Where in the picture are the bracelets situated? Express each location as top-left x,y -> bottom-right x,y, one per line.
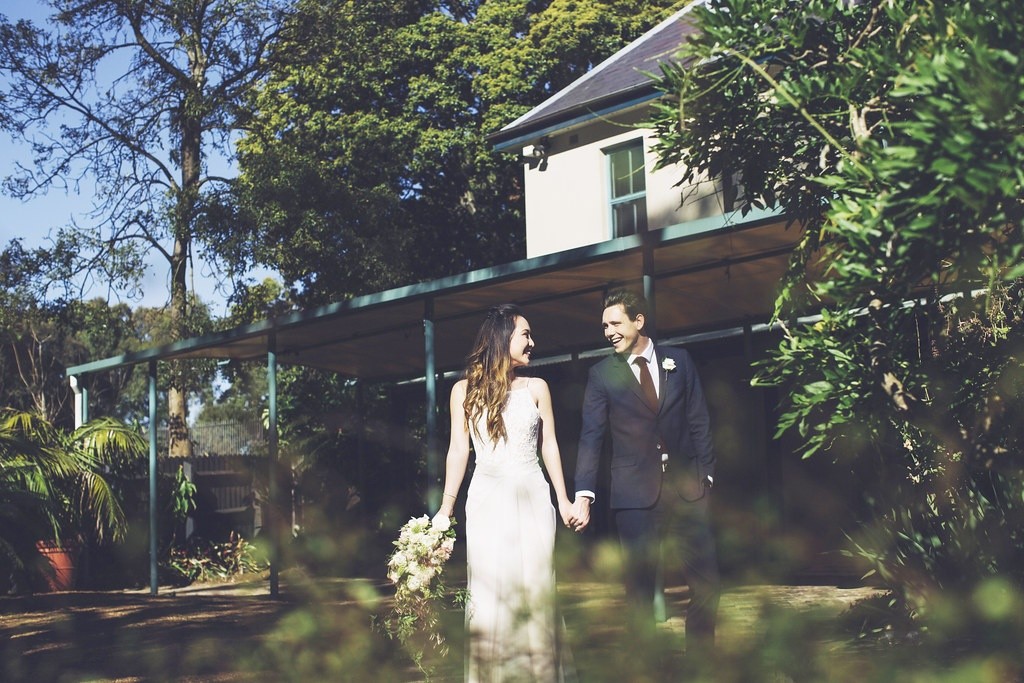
442,492 -> 457,500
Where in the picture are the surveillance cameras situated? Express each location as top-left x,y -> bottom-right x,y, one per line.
522,144 -> 545,158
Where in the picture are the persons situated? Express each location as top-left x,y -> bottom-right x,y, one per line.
432,305 -> 576,682
567,290 -> 722,660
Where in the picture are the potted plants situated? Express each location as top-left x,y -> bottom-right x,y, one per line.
5,317 -> 84,593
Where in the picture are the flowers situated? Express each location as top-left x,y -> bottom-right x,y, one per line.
662,357 -> 676,383
369,513 -> 469,683
